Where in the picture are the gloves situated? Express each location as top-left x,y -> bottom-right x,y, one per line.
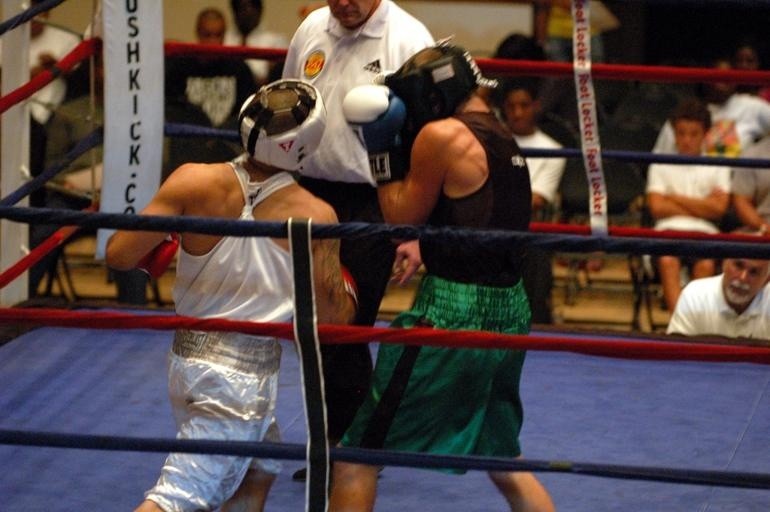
339,262 -> 361,308
343,85 -> 408,187
136,233 -> 180,279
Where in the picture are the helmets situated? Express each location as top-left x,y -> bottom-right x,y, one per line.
239,76 -> 327,174
384,40 -> 503,132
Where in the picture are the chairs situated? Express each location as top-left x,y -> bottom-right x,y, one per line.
538,127 -> 650,332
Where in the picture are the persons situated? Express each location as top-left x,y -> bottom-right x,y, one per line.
322,43 -> 556,511
644,52 -> 769,344
505,2 -> 618,317
28,0 -> 84,208
278,0 -> 439,481
103,78 -> 361,511
164,0 -> 288,169
